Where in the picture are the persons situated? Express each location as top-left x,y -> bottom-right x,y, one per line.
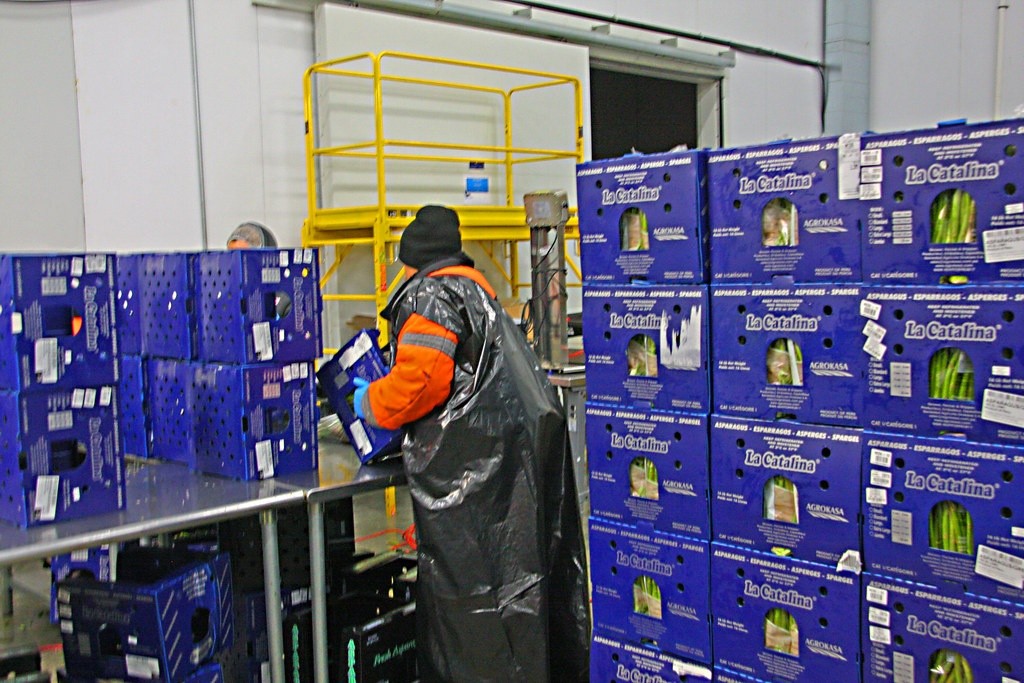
353,206 -> 592,683
227,218 -> 278,253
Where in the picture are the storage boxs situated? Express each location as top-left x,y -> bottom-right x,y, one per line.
0,248 -> 422,683
576,119 -> 1024,683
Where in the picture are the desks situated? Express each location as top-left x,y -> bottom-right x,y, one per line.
0,438 -> 411,683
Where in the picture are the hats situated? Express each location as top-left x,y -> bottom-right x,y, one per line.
398,206 -> 462,270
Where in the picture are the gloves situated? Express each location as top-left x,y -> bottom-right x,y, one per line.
352,378 -> 370,419
